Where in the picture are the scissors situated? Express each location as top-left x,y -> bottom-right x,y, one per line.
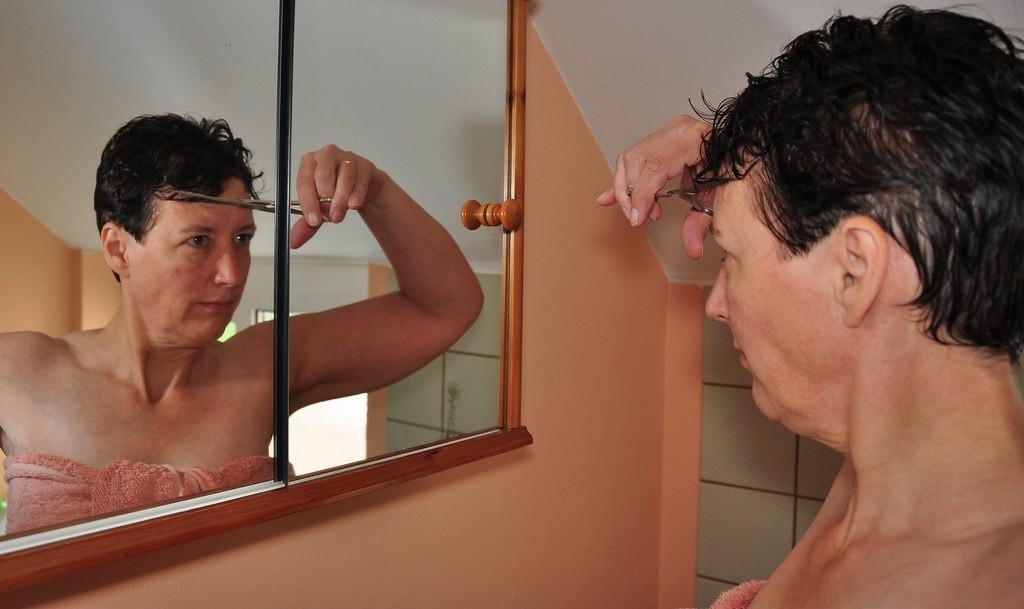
622,163 -> 733,218
166,187 -> 333,221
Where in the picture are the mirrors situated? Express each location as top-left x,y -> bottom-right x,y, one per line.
0,0 -> 536,595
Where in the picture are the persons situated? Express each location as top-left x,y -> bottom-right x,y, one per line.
1,113 -> 484,539
596,4 -> 1024,609
